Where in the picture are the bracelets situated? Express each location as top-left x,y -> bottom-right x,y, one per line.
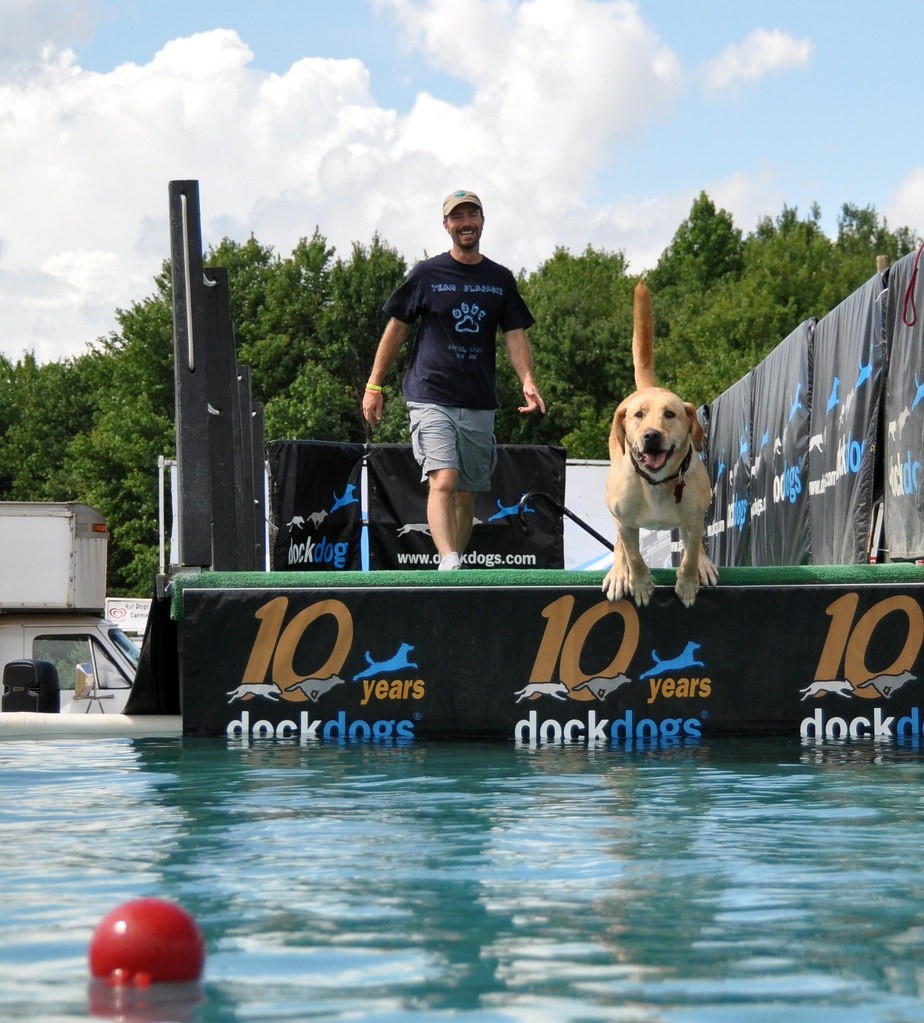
365,389 -> 381,395
367,384 -> 382,390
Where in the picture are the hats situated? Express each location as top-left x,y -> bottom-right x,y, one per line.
443,190 -> 483,218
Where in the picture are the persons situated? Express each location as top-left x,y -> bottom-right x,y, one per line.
362,190 -> 545,571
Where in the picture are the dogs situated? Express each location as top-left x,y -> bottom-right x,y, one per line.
601,277 -> 720,610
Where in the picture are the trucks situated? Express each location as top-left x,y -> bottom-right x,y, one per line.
1,500 -> 143,713
106,597 -> 152,650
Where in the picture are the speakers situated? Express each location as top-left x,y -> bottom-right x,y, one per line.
1,659 -> 60,714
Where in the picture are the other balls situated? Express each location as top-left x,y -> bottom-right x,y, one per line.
88,897 -> 203,989
